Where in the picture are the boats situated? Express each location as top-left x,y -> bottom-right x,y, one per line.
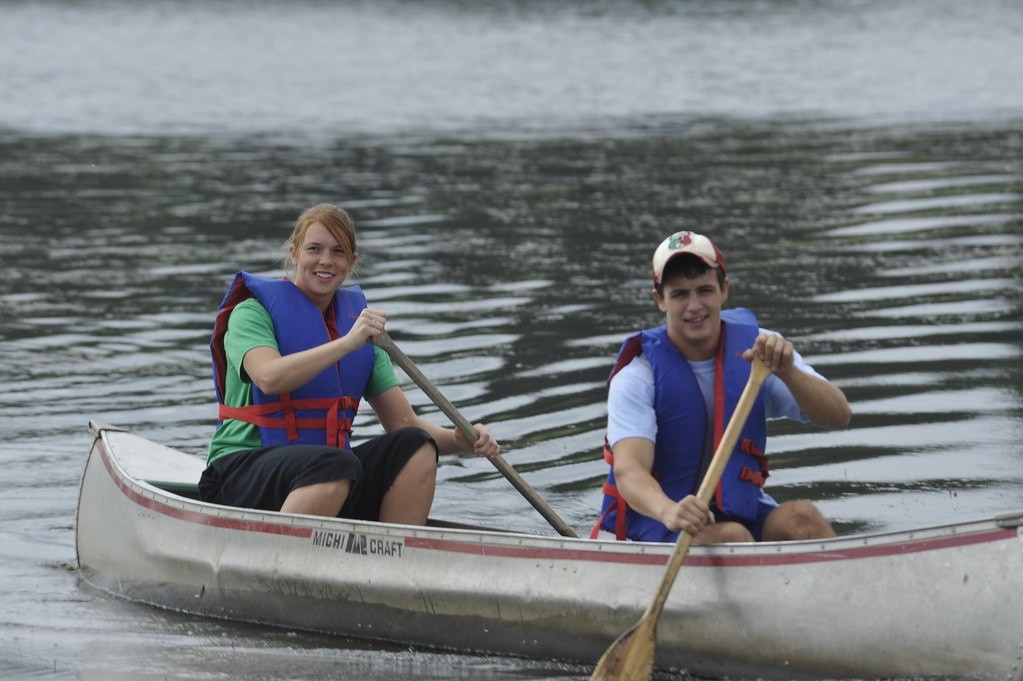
74,420 -> 1022,681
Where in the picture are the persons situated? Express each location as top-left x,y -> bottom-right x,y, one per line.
197,203 -> 501,525
596,231 -> 852,546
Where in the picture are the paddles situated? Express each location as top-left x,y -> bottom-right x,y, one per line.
587,345 -> 776,680
367,324 -> 581,540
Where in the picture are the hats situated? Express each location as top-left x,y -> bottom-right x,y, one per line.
651,231 -> 726,288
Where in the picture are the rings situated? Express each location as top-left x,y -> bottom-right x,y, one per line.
372,320 -> 376,326
